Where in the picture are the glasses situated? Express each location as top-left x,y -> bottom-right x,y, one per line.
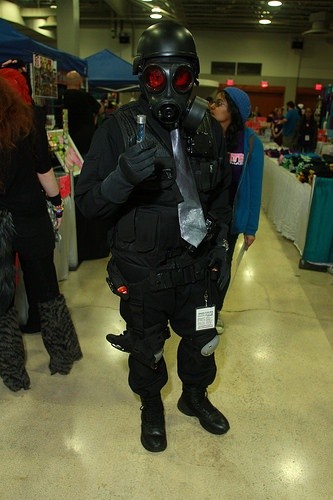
208,99 -> 229,110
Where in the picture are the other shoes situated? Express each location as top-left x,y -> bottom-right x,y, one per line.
19,321 -> 43,334
176,383 -> 230,434
141,391 -> 167,452
214,310 -> 224,334
107,332 -> 130,352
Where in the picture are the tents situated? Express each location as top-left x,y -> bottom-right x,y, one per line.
79,47 -> 146,105
0,18 -> 90,95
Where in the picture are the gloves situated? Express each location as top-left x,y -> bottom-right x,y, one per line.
208,222 -> 230,289
102,135 -> 156,203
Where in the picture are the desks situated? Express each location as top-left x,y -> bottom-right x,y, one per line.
261,141 -> 333,274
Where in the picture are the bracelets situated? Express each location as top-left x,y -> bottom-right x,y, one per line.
46,192 -> 65,213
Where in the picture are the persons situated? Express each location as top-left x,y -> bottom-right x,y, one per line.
1,56 -> 122,135
201,80 -> 333,159
0,67 -> 48,335
0,79 -> 83,394
54,70 -> 106,156
73,20 -> 234,452
207,85 -> 264,336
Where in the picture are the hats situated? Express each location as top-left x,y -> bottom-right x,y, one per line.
223,86 -> 251,122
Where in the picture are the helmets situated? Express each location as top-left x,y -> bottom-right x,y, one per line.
132,21 -> 200,74
